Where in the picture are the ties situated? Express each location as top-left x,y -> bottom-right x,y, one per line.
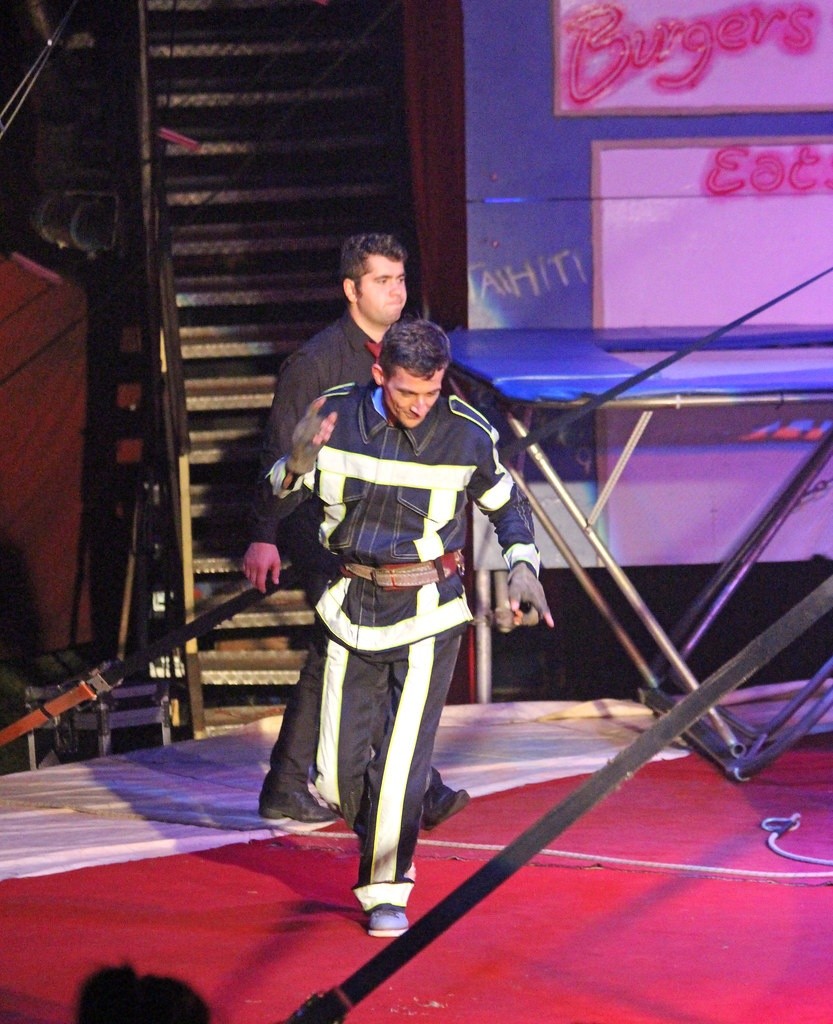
367,341 -> 380,364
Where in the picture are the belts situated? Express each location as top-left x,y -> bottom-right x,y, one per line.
341,547 -> 465,589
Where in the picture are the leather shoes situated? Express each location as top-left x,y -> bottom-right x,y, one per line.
422,785 -> 471,832
257,788 -> 336,822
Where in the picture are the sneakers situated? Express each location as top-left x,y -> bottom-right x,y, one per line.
368,903 -> 408,937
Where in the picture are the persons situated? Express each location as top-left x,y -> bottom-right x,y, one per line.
240,231 -> 471,831
247,318 -> 555,938
